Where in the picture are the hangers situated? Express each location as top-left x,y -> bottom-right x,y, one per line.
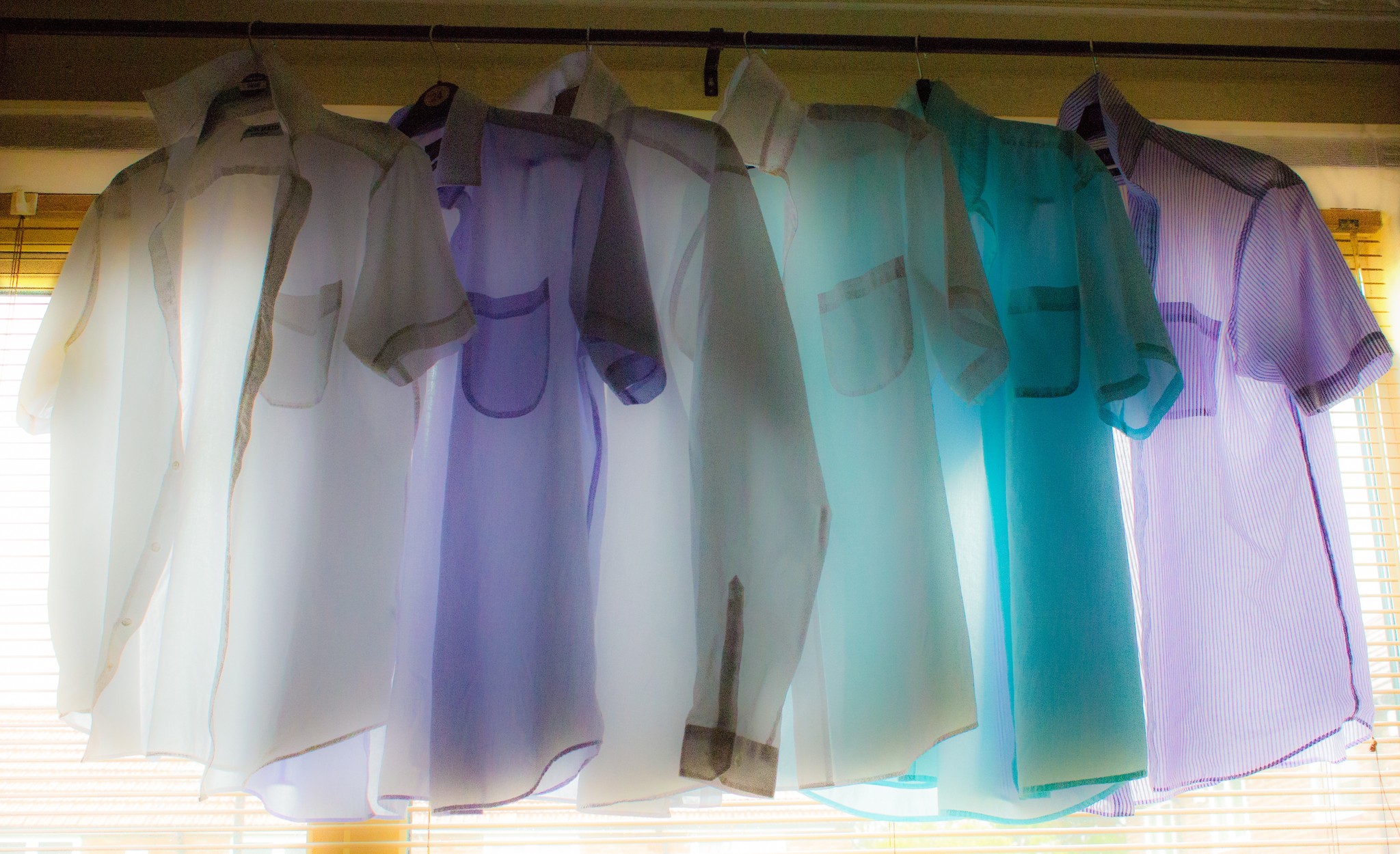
393,22 -> 615,153
112,19 -> 275,183
